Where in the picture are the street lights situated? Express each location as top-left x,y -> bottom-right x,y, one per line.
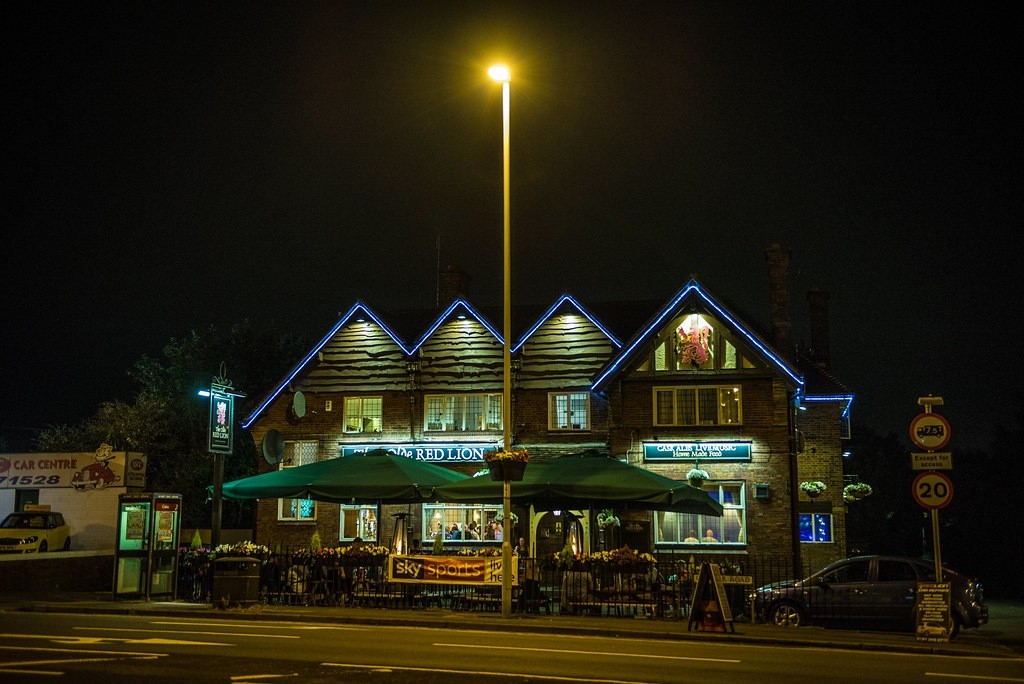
197,362 -> 247,602
487,65 -> 512,620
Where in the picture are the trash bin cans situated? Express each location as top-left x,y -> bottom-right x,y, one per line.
211,557 -> 262,610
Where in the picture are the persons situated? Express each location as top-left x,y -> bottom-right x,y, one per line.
437,520 -> 503,540
684,530 -> 699,542
702,529 -> 719,542
411,539 -> 419,550
352,537 -> 363,546
514,538 -> 530,557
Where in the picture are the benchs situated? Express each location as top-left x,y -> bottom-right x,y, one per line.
570,602 -> 658,619
352,592 -> 552,615
264,590 -> 346,605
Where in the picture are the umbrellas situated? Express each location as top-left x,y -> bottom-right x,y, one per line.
206,448 -> 472,545
435,451 -> 724,557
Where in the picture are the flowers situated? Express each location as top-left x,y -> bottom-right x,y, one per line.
459,547 -> 519,556
553,545 -> 654,562
494,510 -> 519,523
686,469 -> 709,480
842,484 -> 872,500
598,508 -> 620,528
293,543 -> 389,555
801,481 -> 827,490
180,540 -> 269,554
484,447 -> 531,462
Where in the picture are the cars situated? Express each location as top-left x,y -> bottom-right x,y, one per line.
743,555 -> 989,640
0,505 -> 71,554
917,622 -> 947,637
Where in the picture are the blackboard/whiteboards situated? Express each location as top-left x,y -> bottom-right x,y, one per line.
688,563 -> 733,622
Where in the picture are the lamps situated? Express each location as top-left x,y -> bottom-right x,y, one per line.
458,308 -> 466,320
842,449 -> 851,457
356,312 -> 365,323
552,509 -> 561,516
798,404 -> 807,411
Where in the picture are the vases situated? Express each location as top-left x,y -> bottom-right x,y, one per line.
487,460 -> 527,481
604,525 -> 614,531
691,477 -> 703,487
804,489 -> 821,498
544,561 -> 650,573
849,490 -> 868,497
179,551 -> 268,560
501,523 -> 516,529
292,556 -> 386,566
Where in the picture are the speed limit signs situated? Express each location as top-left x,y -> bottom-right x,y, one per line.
912,471 -> 953,509
